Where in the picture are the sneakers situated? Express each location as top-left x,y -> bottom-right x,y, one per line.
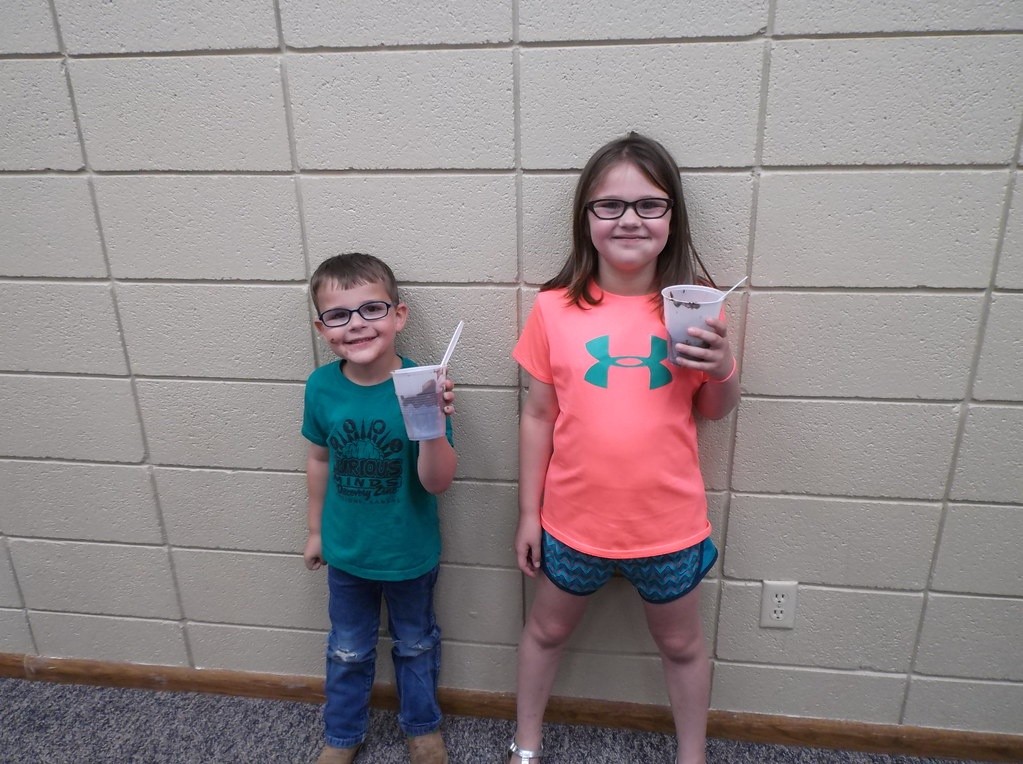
316,745 -> 357,764
405,730 -> 447,764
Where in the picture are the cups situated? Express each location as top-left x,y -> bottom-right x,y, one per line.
391,364 -> 448,441
661,285 -> 726,366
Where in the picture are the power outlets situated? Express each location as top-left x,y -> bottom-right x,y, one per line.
759,579 -> 798,629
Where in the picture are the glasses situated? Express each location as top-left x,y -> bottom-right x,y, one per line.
584,197 -> 673,220
319,301 -> 399,327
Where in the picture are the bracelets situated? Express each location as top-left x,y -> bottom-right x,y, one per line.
707,355 -> 736,385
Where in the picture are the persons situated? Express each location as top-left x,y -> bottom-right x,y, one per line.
302,252 -> 462,764
509,130 -> 740,764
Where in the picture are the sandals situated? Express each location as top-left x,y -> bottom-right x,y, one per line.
507,736 -> 543,764
